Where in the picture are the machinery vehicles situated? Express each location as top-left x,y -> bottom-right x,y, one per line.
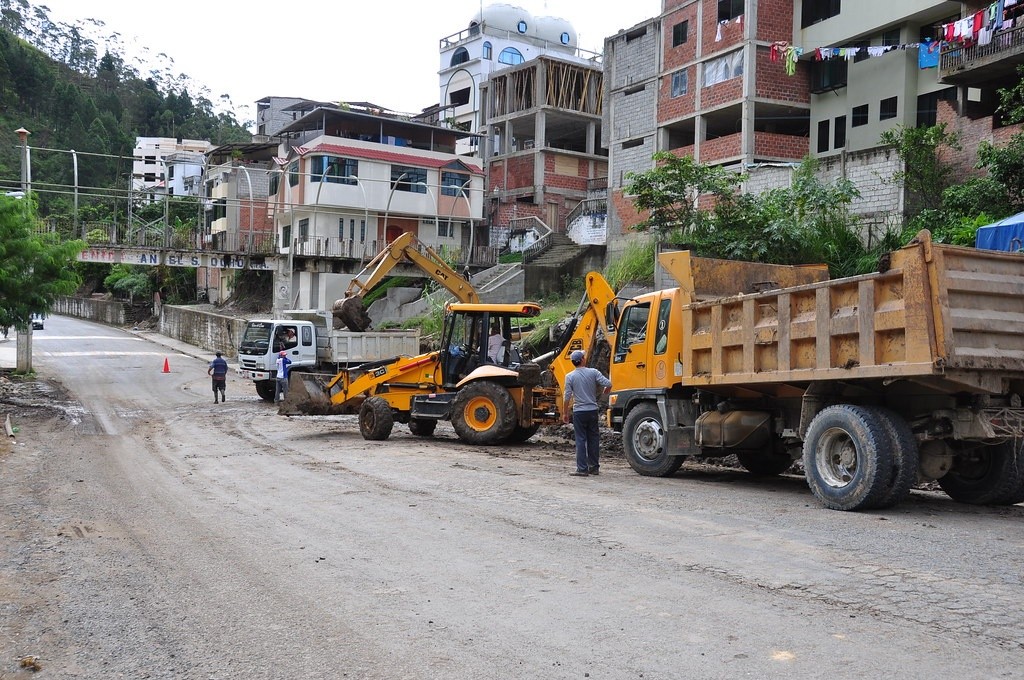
276,231 -> 620,446
237,319 -> 421,400
606,228 -> 1024,512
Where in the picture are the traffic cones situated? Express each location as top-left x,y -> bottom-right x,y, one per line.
160,358 -> 171,374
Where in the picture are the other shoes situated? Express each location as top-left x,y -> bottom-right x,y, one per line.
588,468 -> 599,475
569,470 -> 589,477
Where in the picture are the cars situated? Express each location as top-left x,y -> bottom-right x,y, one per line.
31,314 -> 44,330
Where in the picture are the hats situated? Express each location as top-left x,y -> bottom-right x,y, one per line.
571,350 -> 585,362
280,351 -> 287,355
216,350 -> 221,355
288,328 -> 296,334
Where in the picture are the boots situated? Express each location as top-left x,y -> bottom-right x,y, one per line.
221,389 -> 225,402
213,392 -> 218,404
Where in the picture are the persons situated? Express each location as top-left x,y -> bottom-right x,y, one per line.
563,350 -> 612,476
458,325 -> 507,380
208,350 -> 228,404
274,351 -> 291,403
286,328 -> 296,342
